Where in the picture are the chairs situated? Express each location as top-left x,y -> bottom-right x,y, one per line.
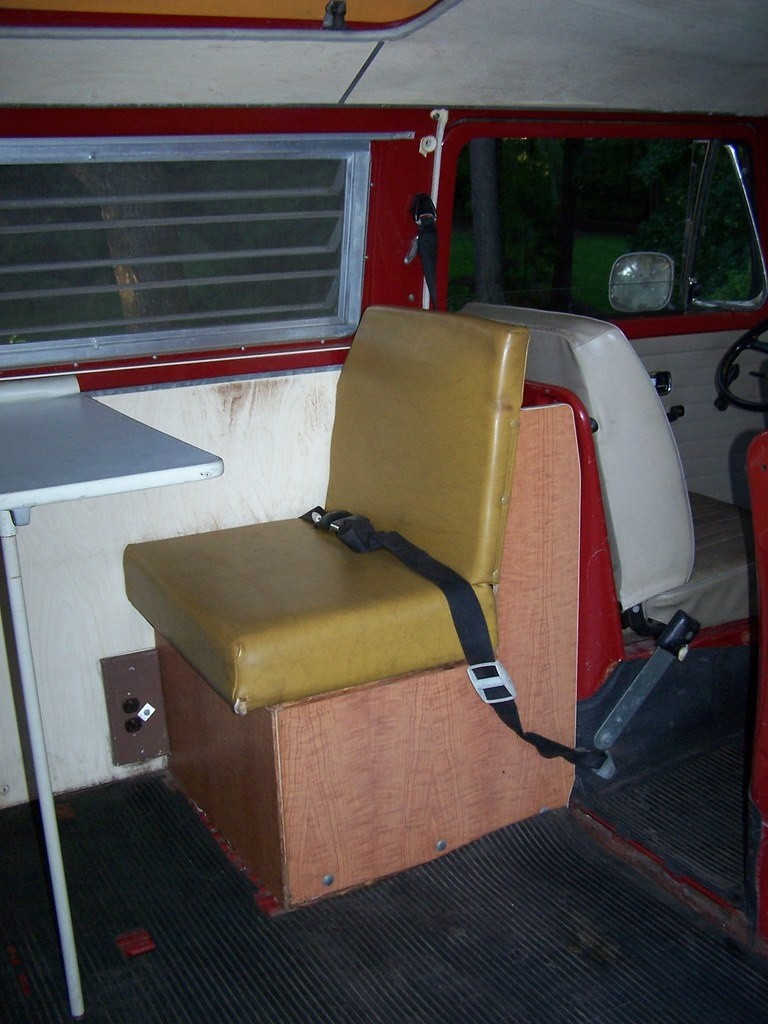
124,301 -> 767,911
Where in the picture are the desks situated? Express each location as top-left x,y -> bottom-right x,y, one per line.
0,393 -> 225,1023
